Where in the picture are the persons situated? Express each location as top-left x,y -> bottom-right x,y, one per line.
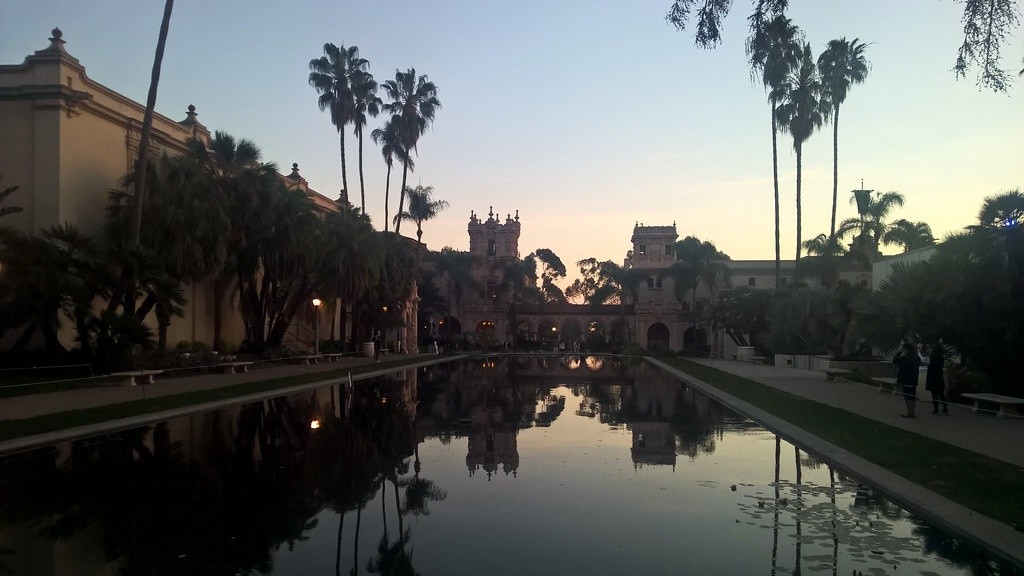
893,342 -> 920,418
540,336 -> 584,353
372,330 -> 383,363
504,338 -> 511,352
925,343 -> 948,415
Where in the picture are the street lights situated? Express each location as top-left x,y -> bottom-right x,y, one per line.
311,390 -> 320,429
552,327 -> 556,352
312,299 -> 321,354
430,317 -> 433,337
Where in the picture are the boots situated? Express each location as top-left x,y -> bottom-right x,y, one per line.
942,403 -> 948,412
931,404 -> 938,413
901,400 -> 916,418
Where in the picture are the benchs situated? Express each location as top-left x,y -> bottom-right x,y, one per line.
379,348 -> 390,354
298,355 -> 323,365
110,369 -> 164,386
218,361 -> 254,374
961,393 -> 1024,419
322,352 -> 345,362
820,368 -> 852,382
873,377 -> 899,394
751,356 -> 767,364
